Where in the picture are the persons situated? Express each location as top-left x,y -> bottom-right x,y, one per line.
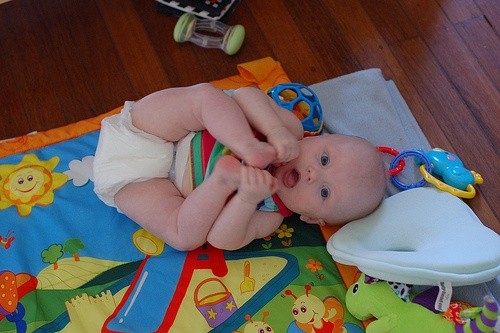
92,82 -> 387,251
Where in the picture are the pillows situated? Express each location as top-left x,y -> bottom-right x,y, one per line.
326,187 -> 500,287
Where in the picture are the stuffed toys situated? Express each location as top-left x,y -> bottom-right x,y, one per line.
345,272 -> 499,333
